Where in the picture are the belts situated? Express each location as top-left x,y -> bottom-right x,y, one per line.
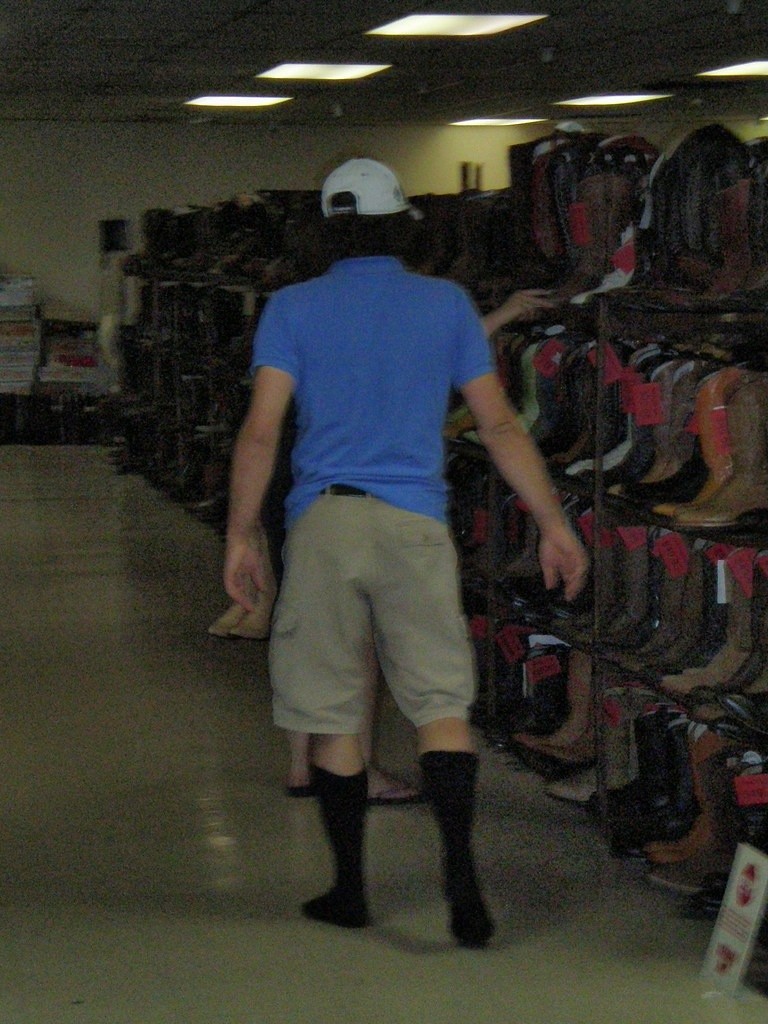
322,483 -> 368,497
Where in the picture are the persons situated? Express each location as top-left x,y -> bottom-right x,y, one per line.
280,288 -> 552,804
224,156 -> 589,952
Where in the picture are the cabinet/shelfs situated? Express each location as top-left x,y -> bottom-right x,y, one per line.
120,265 -> 768,998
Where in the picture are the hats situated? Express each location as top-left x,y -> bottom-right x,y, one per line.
319,154 -> 412,216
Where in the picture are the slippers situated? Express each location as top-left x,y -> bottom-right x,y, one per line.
367,768 -> 426,804
286,777 -> 318,797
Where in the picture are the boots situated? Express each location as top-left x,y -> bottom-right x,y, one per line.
302,762 -> 372,928
106,190 -> 327,641
420,750 -> 495,950
410,132 -> 768,989
